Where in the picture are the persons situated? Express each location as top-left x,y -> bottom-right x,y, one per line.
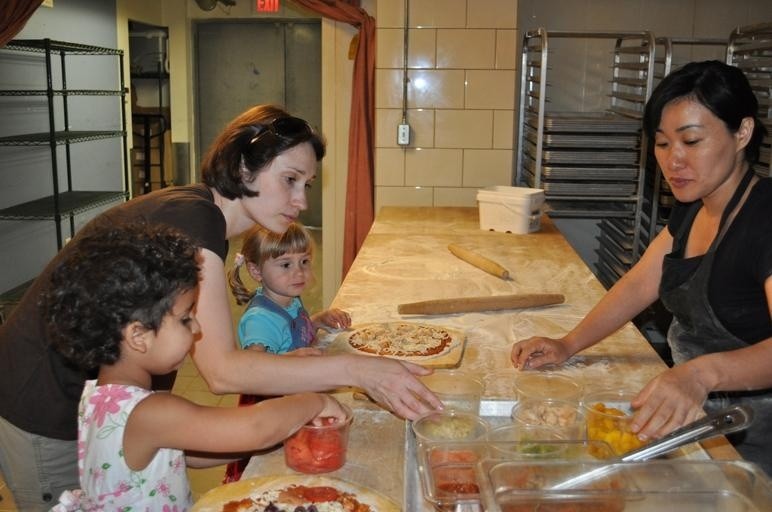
510,58 -> 772,485
226,218 -> 355,482
0,100 -> 448,512
42,213 -> 349,512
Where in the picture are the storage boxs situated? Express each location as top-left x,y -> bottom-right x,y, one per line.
477,183 -> 546,236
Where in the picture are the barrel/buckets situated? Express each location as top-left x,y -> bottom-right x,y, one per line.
474,183 -> 544,235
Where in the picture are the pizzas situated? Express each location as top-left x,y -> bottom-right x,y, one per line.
198,475 -> 380,512
347,321 -> 461,360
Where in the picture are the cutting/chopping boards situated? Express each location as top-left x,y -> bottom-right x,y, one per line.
331,319 -> 469,369
189,473 -> 404,511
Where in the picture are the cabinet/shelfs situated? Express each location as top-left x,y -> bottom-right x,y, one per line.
641,23 -> 772,261
2,35 -> 134,309
519,28 -> 655,294
